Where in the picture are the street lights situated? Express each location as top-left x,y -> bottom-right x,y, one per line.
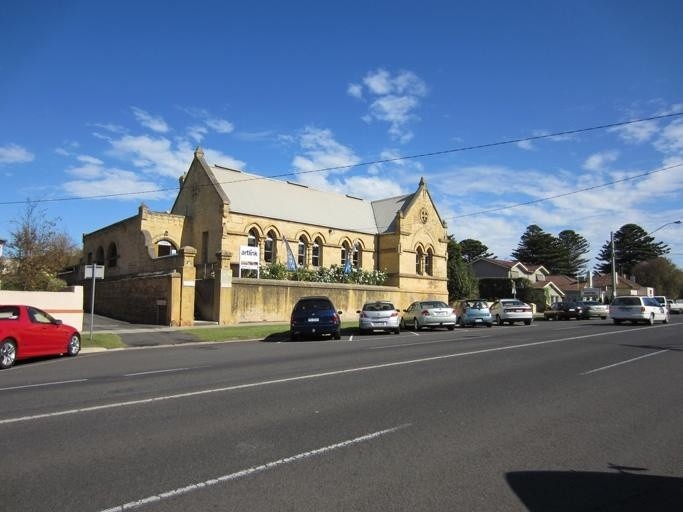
609,220 -> 682,300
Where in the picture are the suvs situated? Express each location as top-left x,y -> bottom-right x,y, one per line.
609,295 -> 682,325
544,301 -> 588,321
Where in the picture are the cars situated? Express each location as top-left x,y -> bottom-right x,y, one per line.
356,301 -> 401,334
401,301 -> 457,331
0,304 -> 82,368
454,299 -> 492,328
489,298 -> 533,325
584,302 -> 609,320
290,296 -> 342,341
